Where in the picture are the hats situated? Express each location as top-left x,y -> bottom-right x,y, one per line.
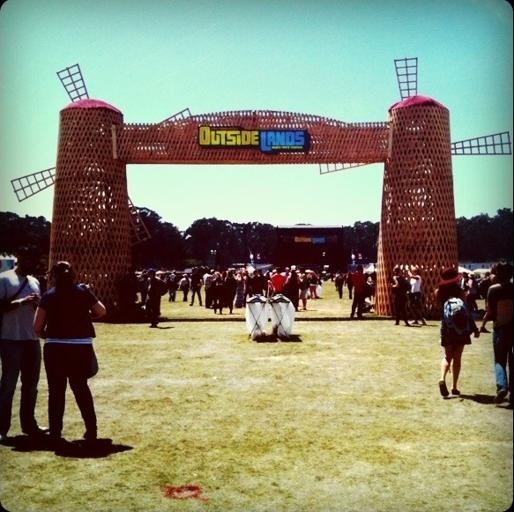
439,267 -> 464,286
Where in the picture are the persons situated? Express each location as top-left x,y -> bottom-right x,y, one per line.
32,260 -> 106,443
434,258 -> 513,406
121,262 -> 428,327
0,249 -> 48,441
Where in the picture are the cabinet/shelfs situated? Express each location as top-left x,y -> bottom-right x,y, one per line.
246,294 -> 291,339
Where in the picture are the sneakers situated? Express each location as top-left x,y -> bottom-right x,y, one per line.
437,380 -> 514,405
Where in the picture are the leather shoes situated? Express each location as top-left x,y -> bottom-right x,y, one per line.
0,431 -> 8,443
23,426 -> 50,434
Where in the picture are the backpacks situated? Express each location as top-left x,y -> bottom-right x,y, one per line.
444,297 -> 472,339
493,298 -> 514,329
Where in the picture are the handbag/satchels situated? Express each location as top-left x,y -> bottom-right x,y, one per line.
88,345 -> 99,378
